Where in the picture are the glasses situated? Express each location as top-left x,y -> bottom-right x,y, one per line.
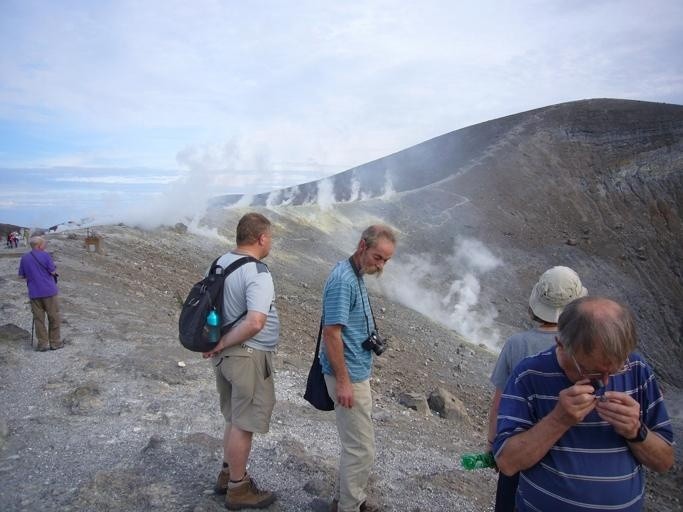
566,346 -> 632,380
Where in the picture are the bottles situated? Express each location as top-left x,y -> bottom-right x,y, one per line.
207,311 -> 220,343
462,453 -> 498,471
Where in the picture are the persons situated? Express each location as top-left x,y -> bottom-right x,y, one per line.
204,211 -> 280,512
18,236 -> 67,352
489,296 -> 676,511
6,232 -> 19,249
317,224 -> 398,511
487,265 -> 591,512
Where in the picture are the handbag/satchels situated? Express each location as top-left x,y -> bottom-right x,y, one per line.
303,309 -> 338,413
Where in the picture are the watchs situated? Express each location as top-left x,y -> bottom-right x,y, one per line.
627,421 -> 647,444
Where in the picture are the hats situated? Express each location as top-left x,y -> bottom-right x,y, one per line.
529,266 -> 588,323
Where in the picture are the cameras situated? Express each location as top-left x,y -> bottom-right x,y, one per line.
362,332 -> 386,356
588,379 -> 605,395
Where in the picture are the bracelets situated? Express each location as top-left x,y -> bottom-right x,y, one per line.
488,439 -> 493,445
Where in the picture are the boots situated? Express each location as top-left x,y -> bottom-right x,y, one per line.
225,478 -> 276,510
214,471 -> 229,493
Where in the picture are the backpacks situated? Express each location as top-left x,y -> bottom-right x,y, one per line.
177,254 -> 273,354
